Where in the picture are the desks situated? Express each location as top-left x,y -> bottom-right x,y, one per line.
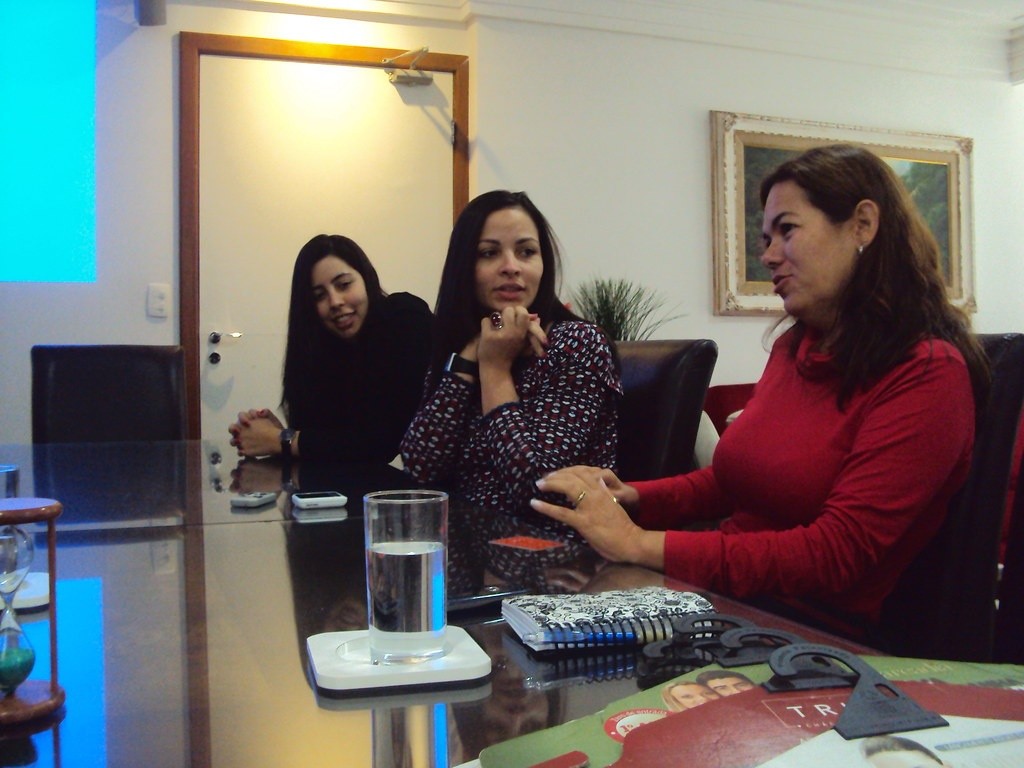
0,444 -> 895,767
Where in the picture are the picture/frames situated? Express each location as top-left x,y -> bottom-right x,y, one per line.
710,110 -> 977,320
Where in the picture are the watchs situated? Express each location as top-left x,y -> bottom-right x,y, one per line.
280,428 -> 296,453
443,353 -> 479,377
280,463 -> 299,493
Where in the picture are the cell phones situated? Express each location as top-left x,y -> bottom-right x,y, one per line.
292,490 -> 347,509
447,582 -> 527,611
291,508 -> 349,523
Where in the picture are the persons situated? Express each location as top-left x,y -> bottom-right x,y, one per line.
228,235 -> 434,490
532,143 -> 992,643
859,736 -> 952,768
696,671 -> 755,698
230,457 -> 394,684
661,681 -> 723,713
399,191 -> 623,548
375,489 -> 612,758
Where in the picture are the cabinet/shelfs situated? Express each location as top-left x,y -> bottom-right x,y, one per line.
0,496 -> 68,723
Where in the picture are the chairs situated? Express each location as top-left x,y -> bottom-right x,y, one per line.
606,340 -> 717,488
875,331 -> 1024,665
31,343 -> 189,444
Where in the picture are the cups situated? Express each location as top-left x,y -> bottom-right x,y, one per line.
365,490 -> 450,665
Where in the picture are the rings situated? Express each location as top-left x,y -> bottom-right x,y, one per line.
573,492 -> 585,506
490,311 -> 503,330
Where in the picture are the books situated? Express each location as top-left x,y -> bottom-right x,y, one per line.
500,585 -> 719,651
